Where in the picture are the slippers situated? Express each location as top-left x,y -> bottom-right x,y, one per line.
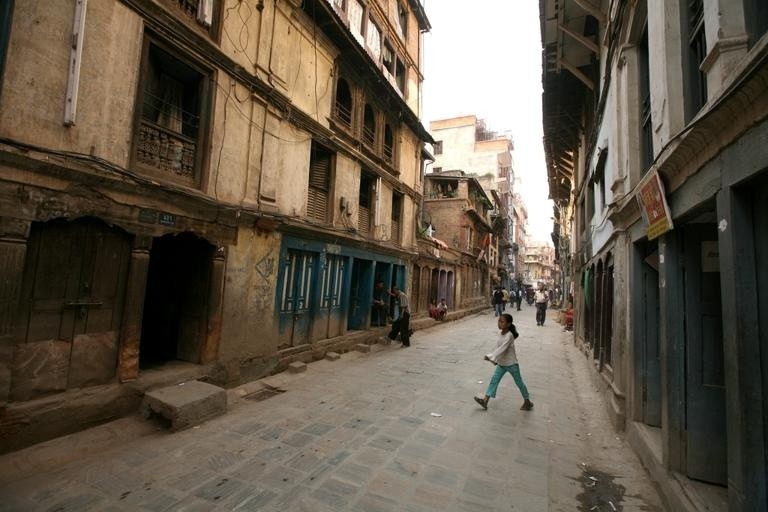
520,403 -> 533,410
474,397 -> 487,408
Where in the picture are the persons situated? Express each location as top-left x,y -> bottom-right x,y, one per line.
429,295 -> 443,320
490,281 -> 574,331
386,285 -> 412,348
437,297 -> 448,317
473,313 -> 534,411
371,279 -> 388,323
